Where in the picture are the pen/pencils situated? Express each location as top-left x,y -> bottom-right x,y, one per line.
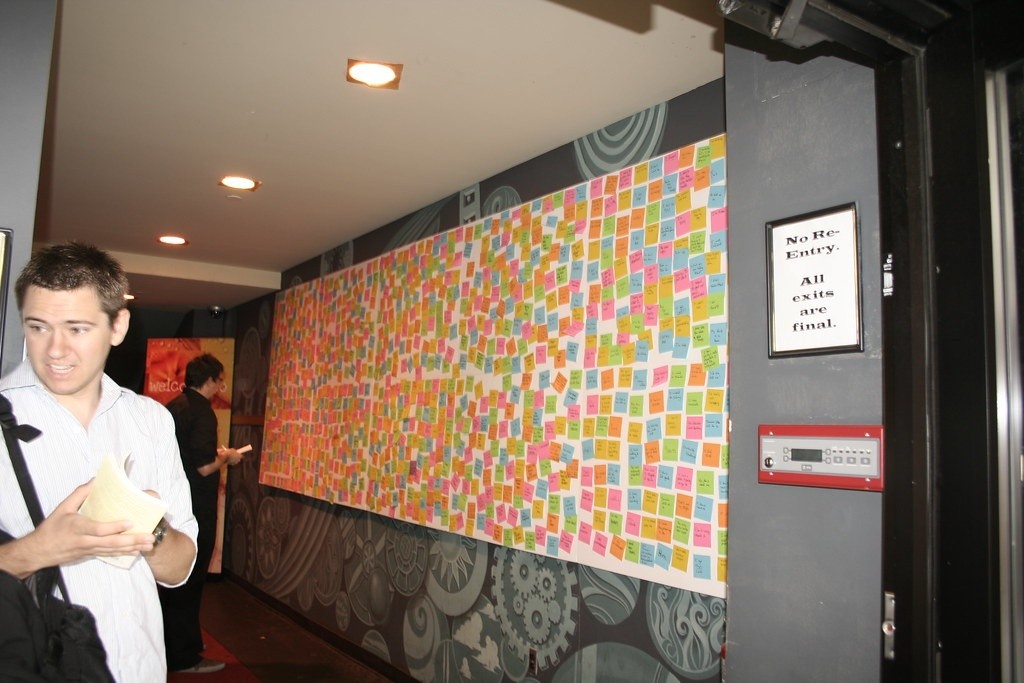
221,444 -> 227,451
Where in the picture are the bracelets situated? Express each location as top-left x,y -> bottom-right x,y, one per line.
155,519 -> 165,544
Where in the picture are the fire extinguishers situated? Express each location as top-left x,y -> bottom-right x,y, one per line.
721,618 -> 726,683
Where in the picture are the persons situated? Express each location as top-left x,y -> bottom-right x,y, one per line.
160,353 -> 246,673
0,241 -> 199,683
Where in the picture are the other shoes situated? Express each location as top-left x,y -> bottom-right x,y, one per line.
176,658 -> 225,673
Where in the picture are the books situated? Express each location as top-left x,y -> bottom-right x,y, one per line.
236,444 -> 252,454
78,453 -> 166,569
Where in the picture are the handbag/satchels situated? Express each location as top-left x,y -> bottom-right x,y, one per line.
0,568 -> 116,683
